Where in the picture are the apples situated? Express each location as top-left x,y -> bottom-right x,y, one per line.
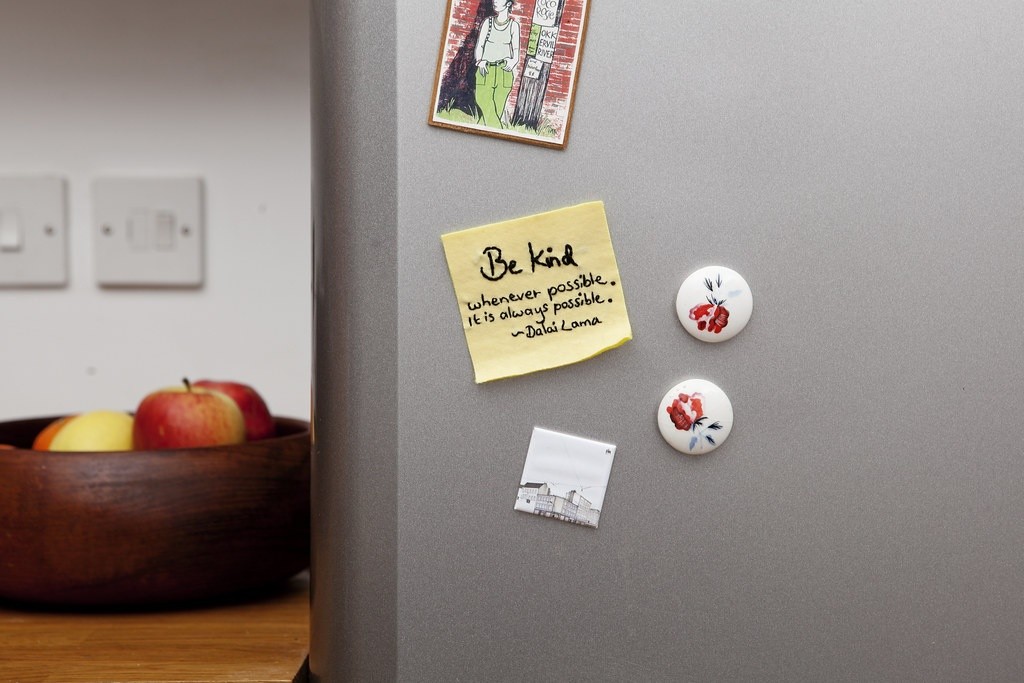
131,378 -> 271,450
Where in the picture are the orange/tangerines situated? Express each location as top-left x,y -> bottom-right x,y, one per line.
31,416 -> 75,453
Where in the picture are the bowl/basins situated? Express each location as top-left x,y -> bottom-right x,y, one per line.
0,415 -> 313,609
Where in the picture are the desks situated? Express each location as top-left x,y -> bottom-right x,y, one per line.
0,572 -> 311,682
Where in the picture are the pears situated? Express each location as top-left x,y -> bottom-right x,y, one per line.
48,410 -> 133,452
